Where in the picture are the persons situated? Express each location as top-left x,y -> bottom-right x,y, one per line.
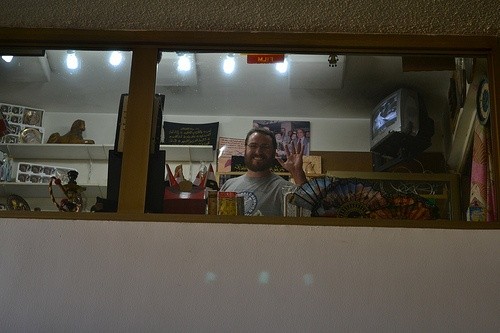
219,127 -> 308,217
254,122 -> 310,155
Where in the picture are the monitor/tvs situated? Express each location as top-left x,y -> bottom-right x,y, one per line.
369,88 -> 419,147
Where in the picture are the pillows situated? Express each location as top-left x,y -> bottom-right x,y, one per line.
163,122 -> 219,150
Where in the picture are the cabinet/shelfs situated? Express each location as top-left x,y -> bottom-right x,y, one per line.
1,141 -> 215,214
220,169 -> 461,224
